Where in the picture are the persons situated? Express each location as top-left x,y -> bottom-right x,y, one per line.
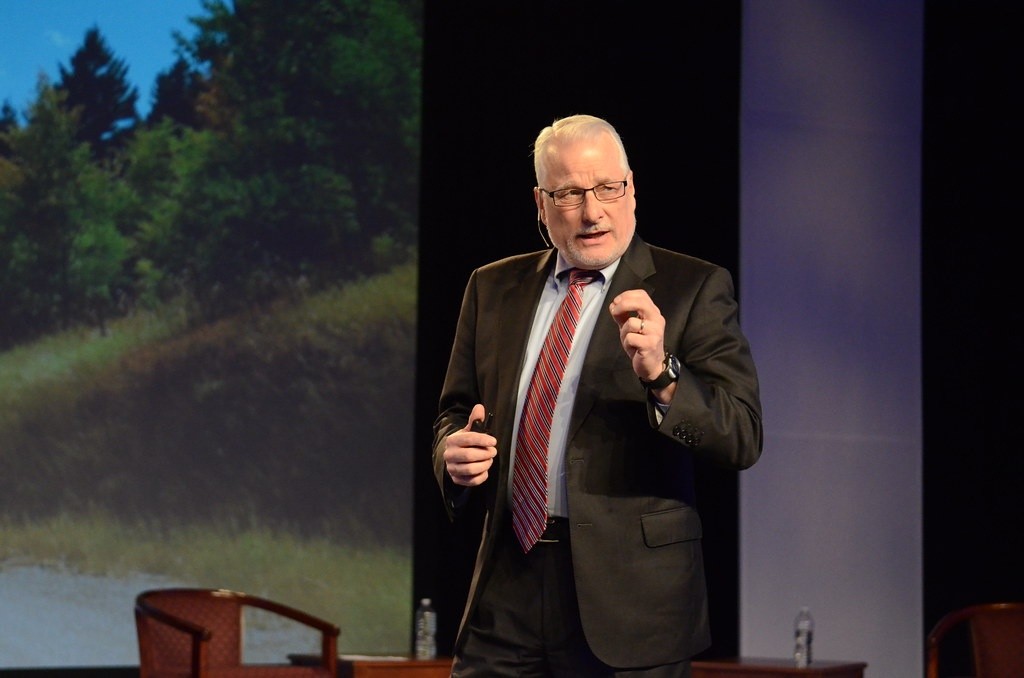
426,115 -> 763,678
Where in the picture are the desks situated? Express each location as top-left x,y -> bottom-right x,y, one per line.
690,656 -> 867,678
289,652 -> 454,678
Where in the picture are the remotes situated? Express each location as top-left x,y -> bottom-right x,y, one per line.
469,412 -> 495,449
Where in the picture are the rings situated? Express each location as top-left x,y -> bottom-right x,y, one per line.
639,318 -> 645,333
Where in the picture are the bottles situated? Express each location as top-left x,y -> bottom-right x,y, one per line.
414,598 -> 438,662
792,604 -> 815,668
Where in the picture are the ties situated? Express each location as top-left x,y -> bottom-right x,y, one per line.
510,270 -> 596,555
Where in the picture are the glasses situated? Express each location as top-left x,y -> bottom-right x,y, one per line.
538,173 -> 629,206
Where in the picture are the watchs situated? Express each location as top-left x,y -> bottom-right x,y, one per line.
638,352 -> 681,391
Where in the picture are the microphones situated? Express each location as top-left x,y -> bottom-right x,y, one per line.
537,207 -> 550,247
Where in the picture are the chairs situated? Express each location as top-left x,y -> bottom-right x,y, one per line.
134,586 -> 341,678
928,602 -> 1024,678
